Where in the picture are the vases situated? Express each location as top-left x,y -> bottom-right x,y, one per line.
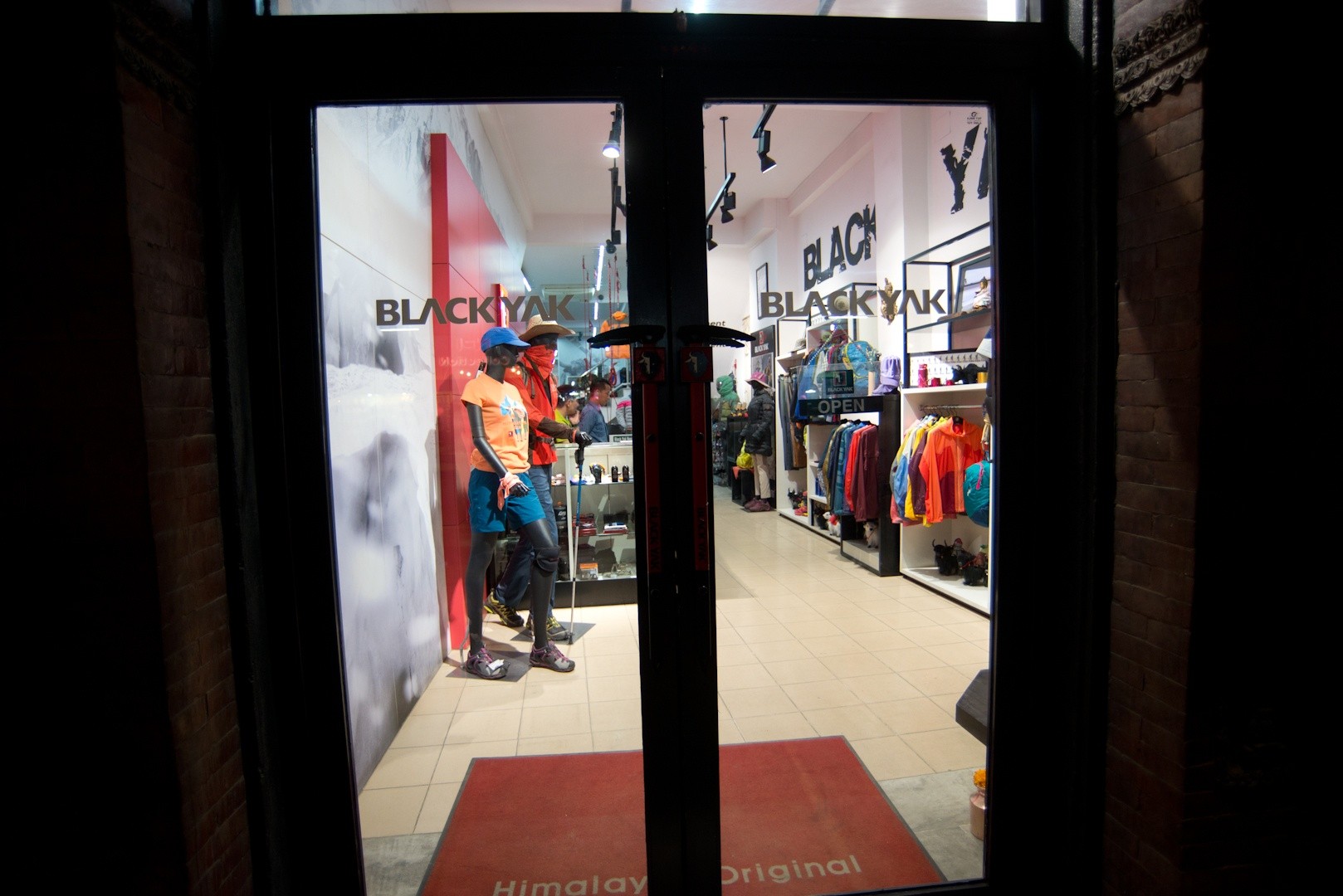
969,791 -> 985,839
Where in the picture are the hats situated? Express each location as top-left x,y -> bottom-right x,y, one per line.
518,314 -> 575,342
480,327 -> 531,352
746,371 -> 769,389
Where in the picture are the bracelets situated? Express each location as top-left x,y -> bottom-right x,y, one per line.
573,429 -> 577,443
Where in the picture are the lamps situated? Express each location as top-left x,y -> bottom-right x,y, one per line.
602,103 -> 777,255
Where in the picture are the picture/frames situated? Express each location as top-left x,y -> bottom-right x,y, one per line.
756,262 -> 769,319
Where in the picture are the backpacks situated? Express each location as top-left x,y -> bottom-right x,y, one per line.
962,450 -> 990,528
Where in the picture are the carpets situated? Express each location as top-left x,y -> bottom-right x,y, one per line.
416,736 -> 949,896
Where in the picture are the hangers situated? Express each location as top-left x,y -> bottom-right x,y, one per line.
922,405 -> 964,425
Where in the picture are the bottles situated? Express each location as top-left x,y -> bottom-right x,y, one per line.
918,364 -> 928,388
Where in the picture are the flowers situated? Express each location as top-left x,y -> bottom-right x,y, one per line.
973,769 -> 986,788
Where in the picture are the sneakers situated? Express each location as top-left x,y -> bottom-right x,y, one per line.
466,645 -> 506,679
795,504 -> 808,516
749,500 -> 770,511
483,588 -> 525,627
528,640 -> 575,672
526,613 -> 568,640
745,497 -> 759,509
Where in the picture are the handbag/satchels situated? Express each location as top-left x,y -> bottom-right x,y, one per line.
736,440 -> 753,469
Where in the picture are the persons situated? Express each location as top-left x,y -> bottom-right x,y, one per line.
555,374 -> 632,443
714,371 -> 776,511
484,313 -> 594,641
460,327 -> 575,679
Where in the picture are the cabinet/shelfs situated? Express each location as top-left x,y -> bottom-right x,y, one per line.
775,282 -> 899,577
494,443 -> 638,584
900,222 -> 992,615
